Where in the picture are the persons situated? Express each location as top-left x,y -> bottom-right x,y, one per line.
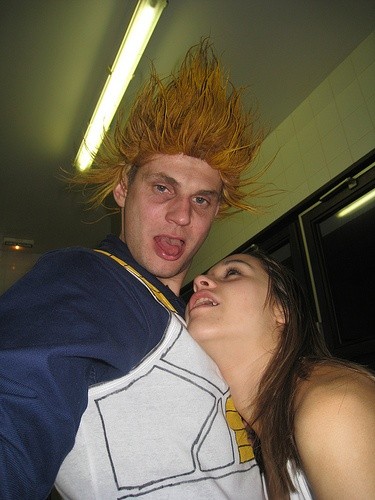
1,38 -> 317,500
187,243 -> 374,500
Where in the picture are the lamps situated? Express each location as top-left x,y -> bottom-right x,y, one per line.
73,0 -> 168,173
